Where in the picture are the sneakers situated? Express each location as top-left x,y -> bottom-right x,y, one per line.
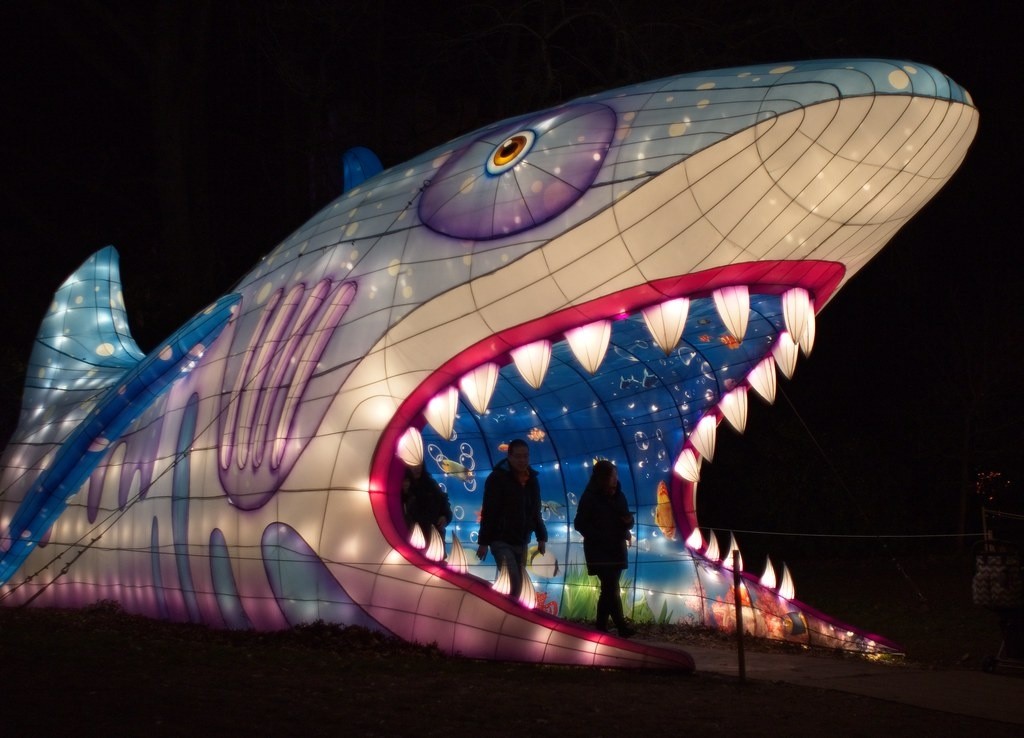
619,625 -> 635,637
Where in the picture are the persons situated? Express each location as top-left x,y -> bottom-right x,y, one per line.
475,440 -> 548,603
399,458 -> 452,561
574,460 -> 639,640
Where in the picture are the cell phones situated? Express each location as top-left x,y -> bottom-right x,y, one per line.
624,511 -> 636,518
